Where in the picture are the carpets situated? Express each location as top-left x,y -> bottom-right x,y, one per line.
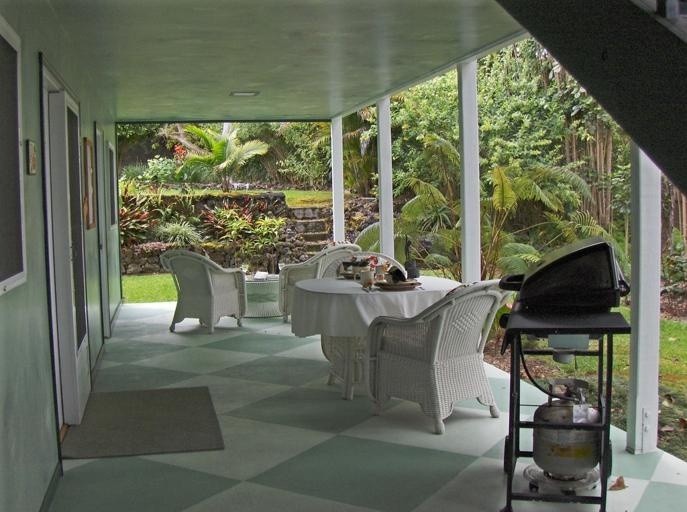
59,386 -> 225,460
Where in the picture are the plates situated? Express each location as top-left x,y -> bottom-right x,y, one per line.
340,271 -> 360,280
375,281 -> 421,290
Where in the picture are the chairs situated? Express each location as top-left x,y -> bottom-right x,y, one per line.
157,247 -> 247,335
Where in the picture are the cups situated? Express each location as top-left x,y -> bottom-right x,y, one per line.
354,270 -> 374,288
342,260 -> 370,274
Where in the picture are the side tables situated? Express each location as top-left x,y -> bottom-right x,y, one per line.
241,273 -> 285,317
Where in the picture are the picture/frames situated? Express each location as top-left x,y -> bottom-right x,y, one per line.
26,139 -> 37,175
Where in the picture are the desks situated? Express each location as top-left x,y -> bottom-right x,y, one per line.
495,310 -> 632,512
